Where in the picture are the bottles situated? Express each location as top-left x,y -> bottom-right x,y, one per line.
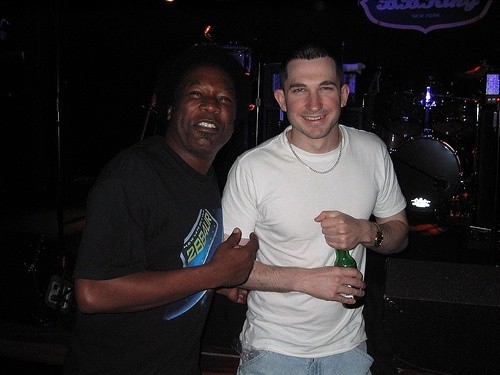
333,248 -> 368,309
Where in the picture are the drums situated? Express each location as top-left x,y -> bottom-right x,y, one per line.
389,131 -> 474,213
449,96 -> 483,124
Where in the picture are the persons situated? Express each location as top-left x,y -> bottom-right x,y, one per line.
58,55 -> 260,375
216,43 -> 410,375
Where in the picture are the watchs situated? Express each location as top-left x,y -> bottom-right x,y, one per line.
367,222 -> 384,249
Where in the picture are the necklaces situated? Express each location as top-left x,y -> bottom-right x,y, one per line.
286,128 -> 342,175
